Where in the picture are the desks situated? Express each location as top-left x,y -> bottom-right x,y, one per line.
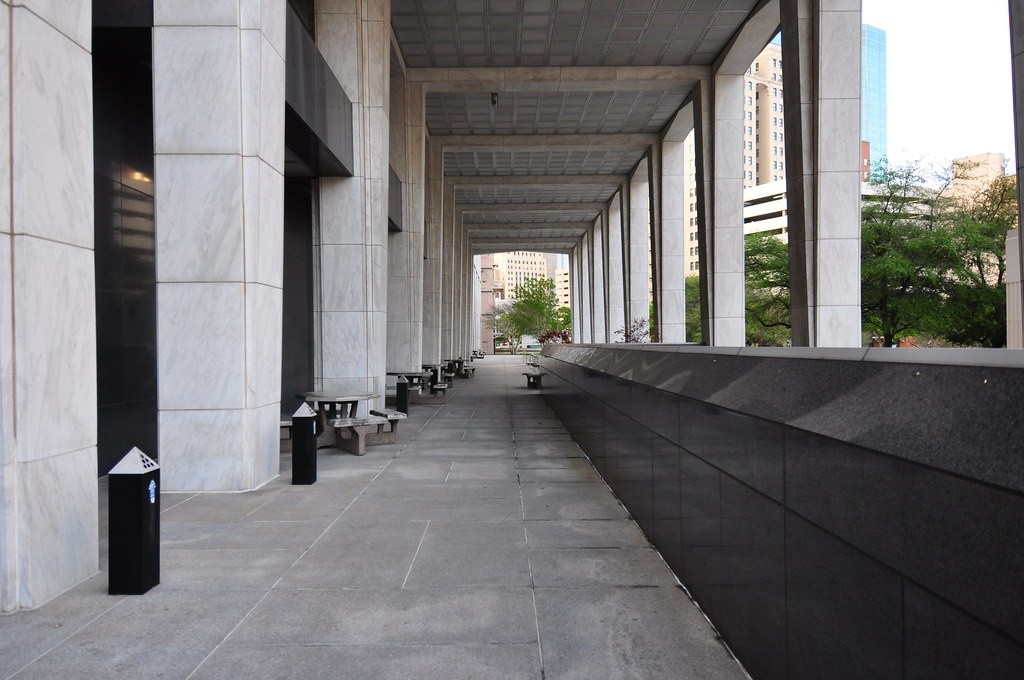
444,359 -> 469,376
295,392 -> 381,448
387,371 -> 433,405
423,364 -> 435,371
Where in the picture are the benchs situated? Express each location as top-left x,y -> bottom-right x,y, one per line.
522,372 -> 545,390
280,410 -> 409,456
385,350 -> 486,408
